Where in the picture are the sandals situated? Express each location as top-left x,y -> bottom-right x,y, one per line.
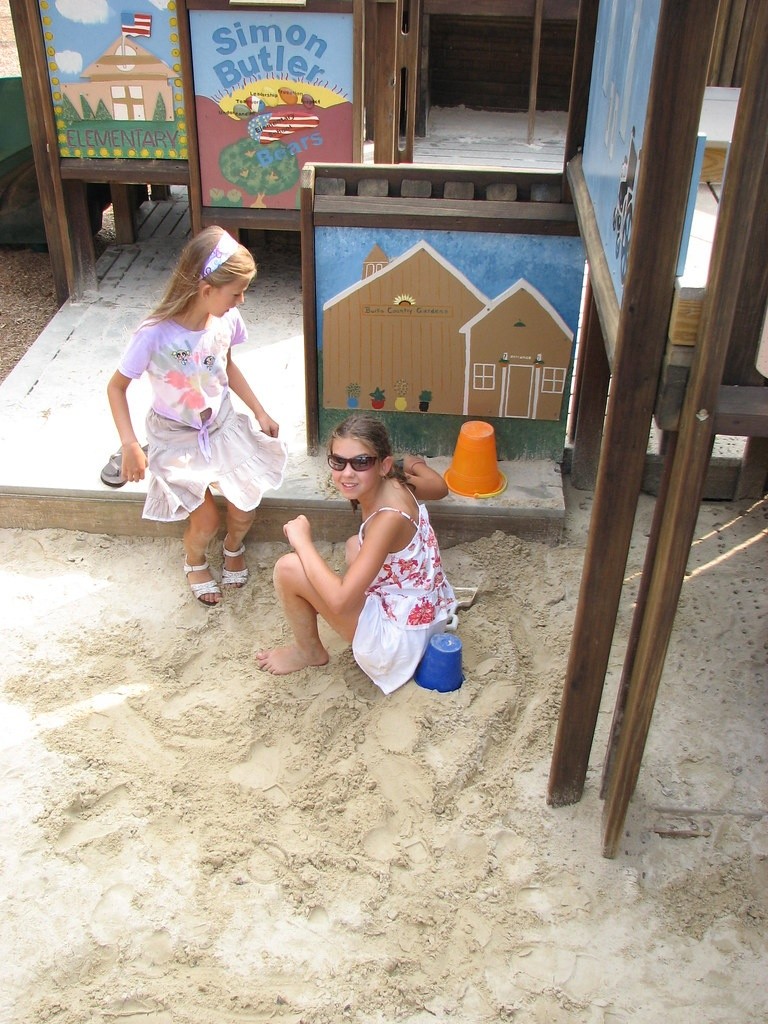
101,445 -> 149,487
222,533 -> 249,588
184,553 -> 222,606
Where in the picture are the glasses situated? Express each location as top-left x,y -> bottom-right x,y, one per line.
327,454 -> 378,472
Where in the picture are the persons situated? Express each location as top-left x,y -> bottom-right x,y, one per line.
106,226 -> 288,609
257,413 -> 458,696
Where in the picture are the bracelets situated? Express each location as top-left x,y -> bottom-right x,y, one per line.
409,460 -> 426,475
121,440 -> 138,448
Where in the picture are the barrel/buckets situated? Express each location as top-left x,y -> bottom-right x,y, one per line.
444,421 -> 508,498
414,633 -> 465,693
